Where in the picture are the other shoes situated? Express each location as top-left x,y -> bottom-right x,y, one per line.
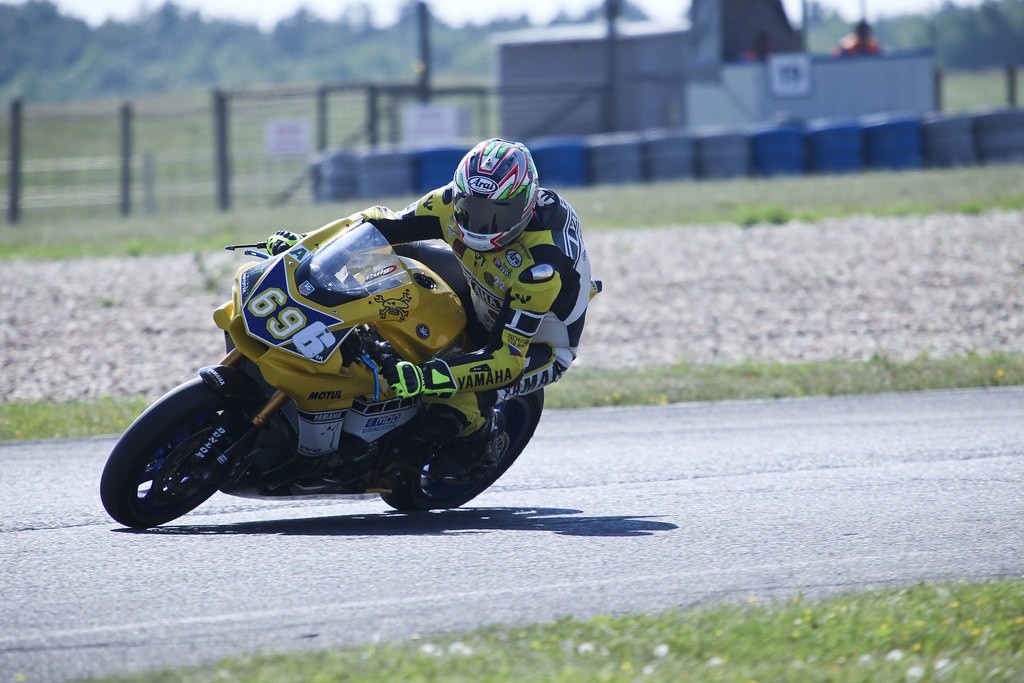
447,408 -> 506,457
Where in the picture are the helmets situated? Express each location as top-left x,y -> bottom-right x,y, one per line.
452,138 -> 539,252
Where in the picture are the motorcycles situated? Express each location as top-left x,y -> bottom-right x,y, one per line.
98,217 -> 603,533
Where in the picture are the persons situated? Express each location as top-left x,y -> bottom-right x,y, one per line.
266,136 -> 591,443
735,36 -> 770,61
831,18 -> 881,56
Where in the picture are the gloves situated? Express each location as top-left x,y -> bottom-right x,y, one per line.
378,358 -> 458,400
266,230 -> 307,256
